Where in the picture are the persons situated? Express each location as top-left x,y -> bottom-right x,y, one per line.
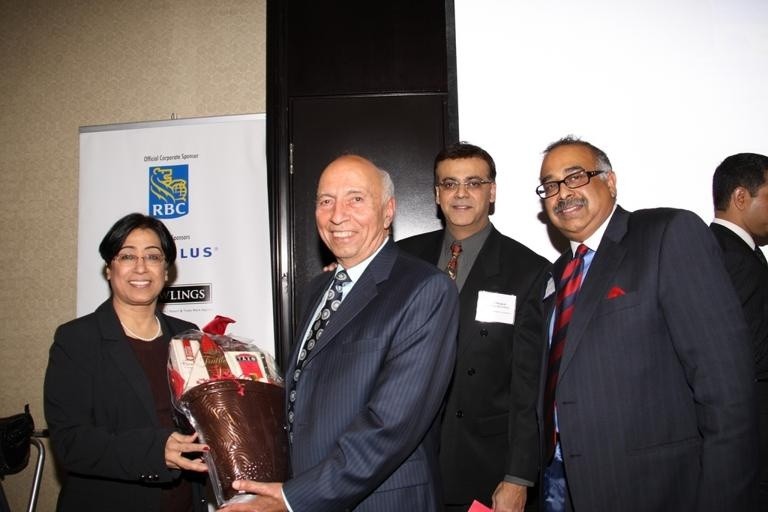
44,212 -> 219,512
537,134 -> 755,512
709,154 -> 768,512
214,155 -> 461,512
322,143 -> 554,511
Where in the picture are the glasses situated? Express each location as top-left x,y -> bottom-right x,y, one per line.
437,179 -> 490,192
536,168 -> 607,199
113,250 -> 168,268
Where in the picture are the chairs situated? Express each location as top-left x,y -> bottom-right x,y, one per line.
0,403 -> 49,512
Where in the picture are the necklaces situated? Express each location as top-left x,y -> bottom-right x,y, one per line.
120,313 -> 161,341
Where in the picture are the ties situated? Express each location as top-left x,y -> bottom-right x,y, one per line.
286,268 -> 350,449
444,243 -> 464,280
534,243 -> 590,468
